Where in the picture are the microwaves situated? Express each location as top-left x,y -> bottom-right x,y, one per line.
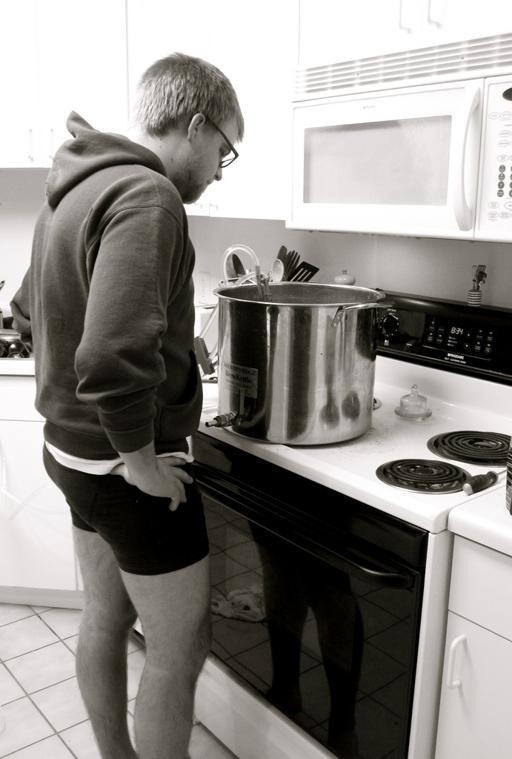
285,73 -> 512,243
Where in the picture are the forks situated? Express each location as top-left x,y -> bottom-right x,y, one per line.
282,250 -> 299,281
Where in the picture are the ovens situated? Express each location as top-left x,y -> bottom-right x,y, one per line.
174,432 -> 429,759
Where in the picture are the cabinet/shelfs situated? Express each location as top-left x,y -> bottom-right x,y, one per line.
434,534 -> 511,759
0,375 -> 86,611
127,0 -> 300,219
0,0 -> 126,168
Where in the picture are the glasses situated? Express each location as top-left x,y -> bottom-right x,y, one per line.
207,114 -> 238,170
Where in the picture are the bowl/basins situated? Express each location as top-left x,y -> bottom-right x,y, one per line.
0,338 -> 30,358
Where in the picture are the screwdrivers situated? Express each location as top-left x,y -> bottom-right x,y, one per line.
463,469 -> 508,495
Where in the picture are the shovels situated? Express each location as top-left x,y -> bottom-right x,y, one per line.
287,261 -> 320,282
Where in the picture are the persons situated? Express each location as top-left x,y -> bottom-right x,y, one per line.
208,439 -> 367,759
9,48 -> 252,758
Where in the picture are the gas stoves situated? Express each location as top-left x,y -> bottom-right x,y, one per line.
197,380 -> 512,534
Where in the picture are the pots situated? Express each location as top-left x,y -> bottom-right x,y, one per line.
213,282 -> 398,445
